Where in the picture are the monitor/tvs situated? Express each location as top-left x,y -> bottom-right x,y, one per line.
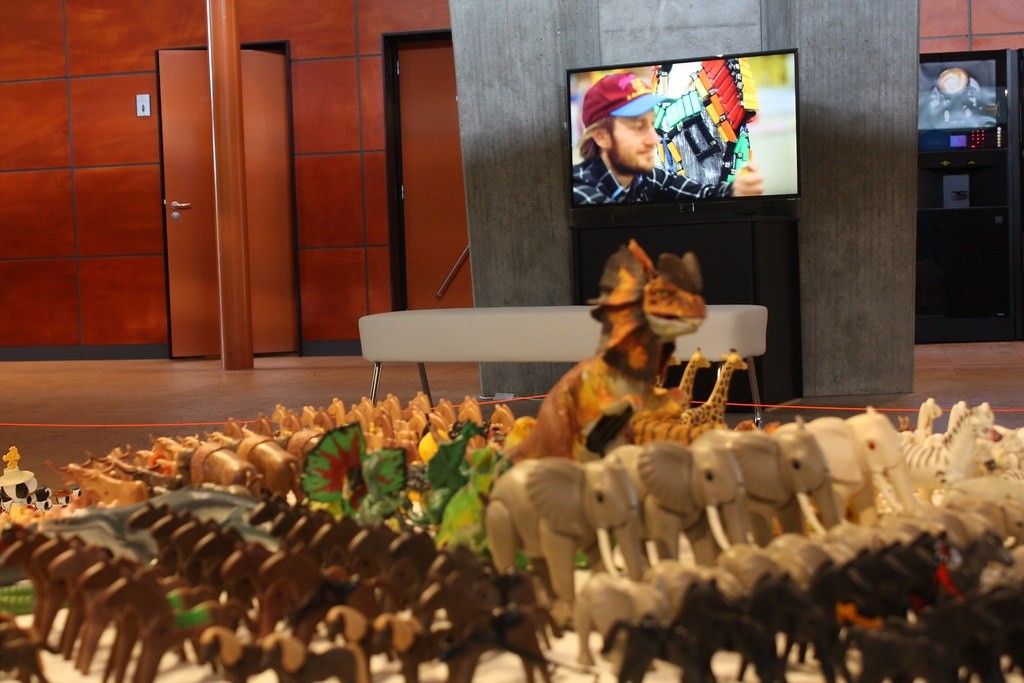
567,48 -> 801,210
918,49 -> 1008,151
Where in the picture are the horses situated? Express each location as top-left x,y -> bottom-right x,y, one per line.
603,529 -> 1024,682
0,488 -> 556,683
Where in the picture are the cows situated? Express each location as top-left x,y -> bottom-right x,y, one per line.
46,415 -> 325,517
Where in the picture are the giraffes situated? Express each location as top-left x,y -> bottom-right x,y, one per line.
654,345 -> 748,428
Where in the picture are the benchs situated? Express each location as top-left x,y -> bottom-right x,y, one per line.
358,304 -> 768,418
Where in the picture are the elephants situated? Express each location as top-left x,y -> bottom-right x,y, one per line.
481,413 -> 937,667
